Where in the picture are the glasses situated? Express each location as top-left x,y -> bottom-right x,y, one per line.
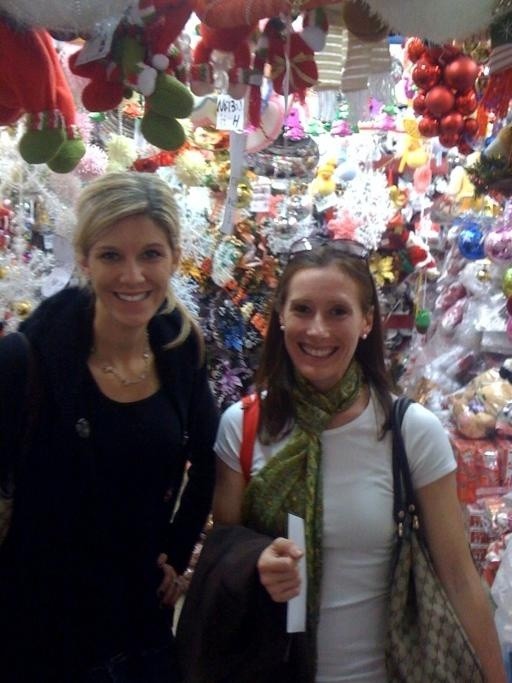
288,236 -> 370,259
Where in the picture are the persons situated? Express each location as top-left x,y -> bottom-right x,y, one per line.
0,173 -> 220,683
211,239 -> 507,683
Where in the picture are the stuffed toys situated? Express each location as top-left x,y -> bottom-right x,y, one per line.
1,0 -> 511,438
0,0 -> 512,439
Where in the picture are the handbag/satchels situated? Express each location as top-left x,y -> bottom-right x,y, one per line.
382,394 -> 499,683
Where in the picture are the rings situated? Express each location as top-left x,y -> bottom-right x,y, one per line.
171,574 -> 179,587
